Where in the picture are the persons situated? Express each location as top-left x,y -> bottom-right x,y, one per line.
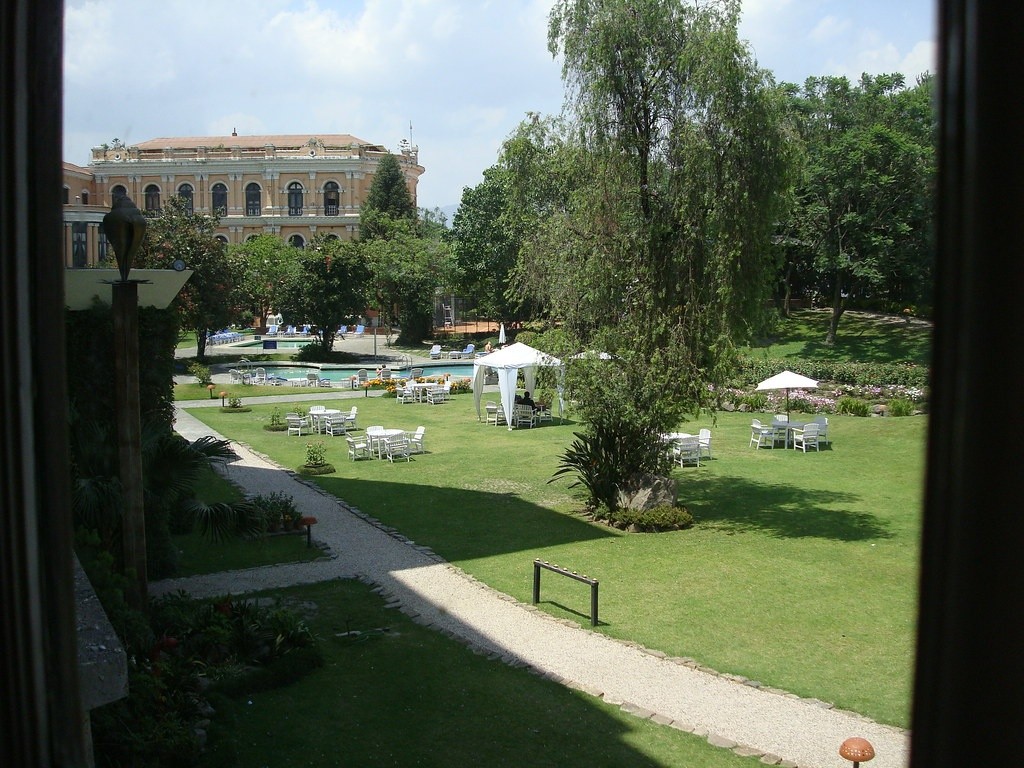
517,392 -> 535,428
485,341 -> 493,353
377,364 -> 392,376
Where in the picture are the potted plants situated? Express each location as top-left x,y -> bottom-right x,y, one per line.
262,490 -> 303,532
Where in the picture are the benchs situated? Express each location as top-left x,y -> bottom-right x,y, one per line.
318,330 -> 323,338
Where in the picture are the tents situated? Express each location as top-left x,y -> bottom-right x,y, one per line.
471,341 -> 568,426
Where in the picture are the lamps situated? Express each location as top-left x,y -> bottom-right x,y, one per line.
172,259 -> 186,271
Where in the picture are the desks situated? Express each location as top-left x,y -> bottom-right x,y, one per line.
364,429 -> 404,460
308,409 -> 340,434
294,332 -> 301,337
406,383 -> 438,403
277,331 -> 285,337
289,377 -> 308,387
449,351 -> 461,359
657,433 -> 691,464
513,404 -> 544,426
341,378 -> 351,387
347,332 -> 354,337
271,379 -> 284,386
770,420 -> 809,450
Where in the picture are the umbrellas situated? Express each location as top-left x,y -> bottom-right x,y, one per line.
754,370 -> 819,446
568,349 -> 615,359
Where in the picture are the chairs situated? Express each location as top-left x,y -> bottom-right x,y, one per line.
749,419 -> 776,450
430,344 -> 442,360
772,414 -> 793,444
353,325 -> 365,338
366,426 -> 386,456
229,369 -> 248,383
253,367 -> 287,386
337,406 -> 359,433
441,351 -> 449,359
205,328 -> 245,346
673,435 -> 700,468
284,325 -> 295,338
310,406 -> 326,425
459,343 -> 475,359
319,378 -> 330,387
307,373 -> 320,387
384,431 -> 410,463
427,384 -> 445,405
300,326 -> 311,338
376,367 -> 391,380
285,413 -> 310,437
346,432 -> 371,462
337,325 -> 347,337
354,369 -> 368,387
396,384 -> 413,405
438,381 -> 452,401
791,423 -> 820,453
691,428 -> 712,461
485,400 -> 508,426
813,417 -> 828,445
474,352 -> 489,359
406,380 -> 420,401
402,426 -> 427,456
266,325 -> 279,337
517,403 -> 537,428
324,412 -> 346,437
536,393 -> 554,423
399,368 -> 424,379
